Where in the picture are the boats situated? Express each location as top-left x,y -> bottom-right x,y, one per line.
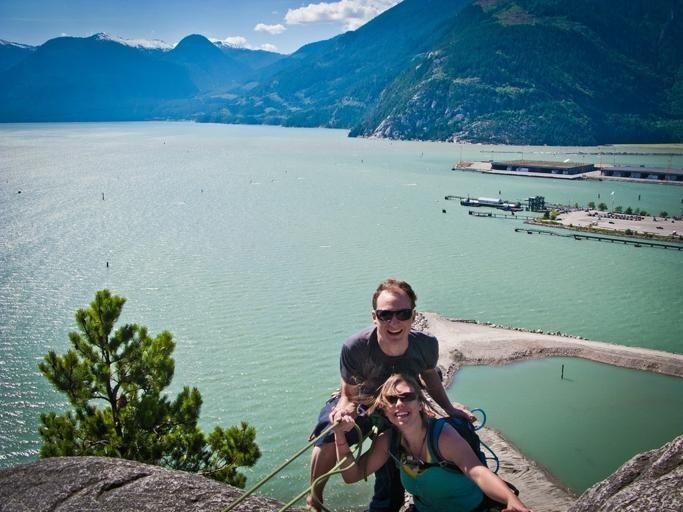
459,194 -> 525,213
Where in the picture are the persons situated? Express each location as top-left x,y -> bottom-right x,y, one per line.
331,372 -> 533,512
305,277 -> 477,510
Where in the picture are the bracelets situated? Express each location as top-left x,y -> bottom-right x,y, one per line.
334,441 -> 348,447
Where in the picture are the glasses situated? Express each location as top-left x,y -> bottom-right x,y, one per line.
385,389 -> 417,404
374,307 -> 413,321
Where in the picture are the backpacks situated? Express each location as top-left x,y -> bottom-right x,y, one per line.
391,414 -> 487,473
